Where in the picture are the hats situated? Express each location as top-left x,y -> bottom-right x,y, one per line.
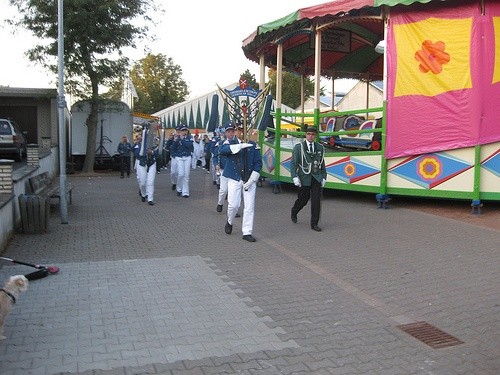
175,125 -> 181,130
225,124 -> 234,131
219,127 -> 225,132
305,126 -> 318,132
180,124 -> 189,130
235,122 -> 244,128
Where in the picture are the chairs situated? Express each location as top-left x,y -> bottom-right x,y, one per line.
342,120 -> 378,149
319,118 -> 336,142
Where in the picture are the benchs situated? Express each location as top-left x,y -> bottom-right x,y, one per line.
29,171 -> 74,208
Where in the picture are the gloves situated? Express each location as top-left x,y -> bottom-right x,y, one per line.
230,143 -> 253,154
321,178 -> 325,187
244,170 -> 260,191
293,177 -> 302,188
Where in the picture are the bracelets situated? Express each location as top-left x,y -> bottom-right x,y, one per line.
152,150 -> 154,155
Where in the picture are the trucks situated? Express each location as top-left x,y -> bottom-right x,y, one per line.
69,98 -> 133,171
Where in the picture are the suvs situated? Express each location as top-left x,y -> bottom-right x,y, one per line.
0,118 -> 27,162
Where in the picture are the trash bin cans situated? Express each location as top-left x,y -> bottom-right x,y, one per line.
19,193 -> 50,235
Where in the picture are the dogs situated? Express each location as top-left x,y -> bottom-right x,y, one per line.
0,275 -> 30,340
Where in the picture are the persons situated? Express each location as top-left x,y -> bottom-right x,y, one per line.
291,126 -> 327,231
132,124 -> 241,218
117,135 -> 132,178
220,122 -> 262,242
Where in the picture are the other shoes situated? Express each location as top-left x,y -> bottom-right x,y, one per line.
127,174 -> 130,177
207,170 -> 210,174
177,193 -> 181,196
142,197 -> 145,202
291,207 -> 298,223
119,175 -> 124,178
172,184 -> 176,190
225,224 -> 232,234
139,190 -> 141,196
148,201 -> 153,205
242,235 -> 256,241
236,213 -> 240,218
184,195 -> 188,198
217,185 -> 220,189
212,181 -> 216,185
311,225 -> 322,231
217,205 -> 223,212
